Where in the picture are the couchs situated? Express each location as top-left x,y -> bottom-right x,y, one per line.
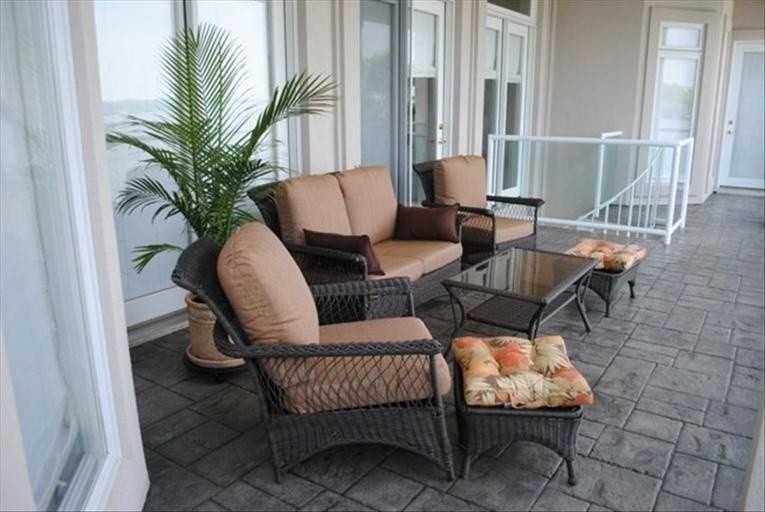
248,166 -> 464,319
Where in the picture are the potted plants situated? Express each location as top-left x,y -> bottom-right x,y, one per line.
107,20 -> 341,376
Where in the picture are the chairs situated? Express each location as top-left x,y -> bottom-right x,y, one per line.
172,222 -> 457,484
412,155 -> 546,266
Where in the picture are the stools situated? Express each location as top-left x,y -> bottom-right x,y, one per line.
562,238 -> 648,319
448,334 -> 593,486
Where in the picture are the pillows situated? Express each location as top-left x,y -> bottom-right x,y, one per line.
398,203 -> 460,243
563,238 -> 647,271
451,335 -> 594,409
432,156 -> 488,219
303,229 -> 385,276
333,168 -> 399,247
217,223 -> 320,390
274,175 -> 353,246
